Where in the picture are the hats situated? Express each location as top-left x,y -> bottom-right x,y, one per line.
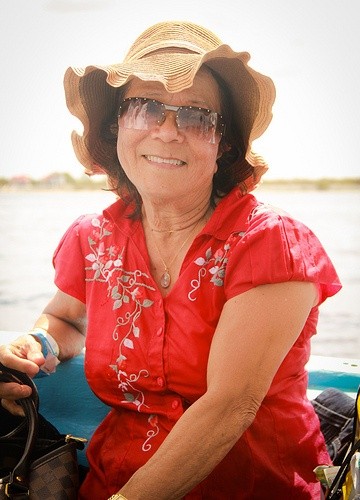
63,21 -> 277,198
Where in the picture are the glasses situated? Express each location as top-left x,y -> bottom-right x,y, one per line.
116,97 -> 224,145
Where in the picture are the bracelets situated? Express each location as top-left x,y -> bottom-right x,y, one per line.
108,493 -> 128,500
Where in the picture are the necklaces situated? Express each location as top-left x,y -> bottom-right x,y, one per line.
146,220 -> 201,287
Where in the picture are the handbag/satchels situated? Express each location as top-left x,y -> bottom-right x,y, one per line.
0,362 -> 88,500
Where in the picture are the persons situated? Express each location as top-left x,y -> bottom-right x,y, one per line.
0,19 -> 343,500
